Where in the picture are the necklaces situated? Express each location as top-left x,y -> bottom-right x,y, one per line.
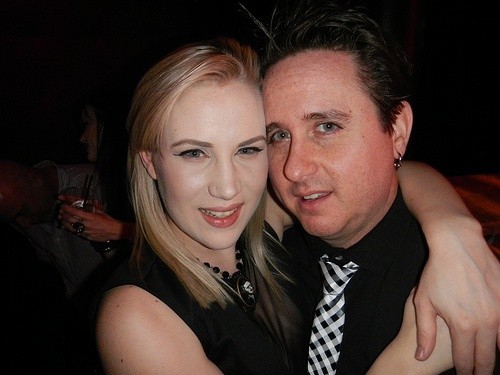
196,249 -> 256,309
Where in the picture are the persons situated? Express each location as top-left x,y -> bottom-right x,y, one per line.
261,2 -> 498,374
241,280 -> 255,300
91,34 -> 500,375
57,81 -> 137,256
0,158 -> 104,317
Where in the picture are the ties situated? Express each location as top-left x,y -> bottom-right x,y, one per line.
306,254 -> 358,375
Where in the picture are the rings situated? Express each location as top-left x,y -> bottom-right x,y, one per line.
72,222 -> 85,235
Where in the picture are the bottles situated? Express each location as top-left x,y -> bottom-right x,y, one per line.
104,240 -> 121,265
